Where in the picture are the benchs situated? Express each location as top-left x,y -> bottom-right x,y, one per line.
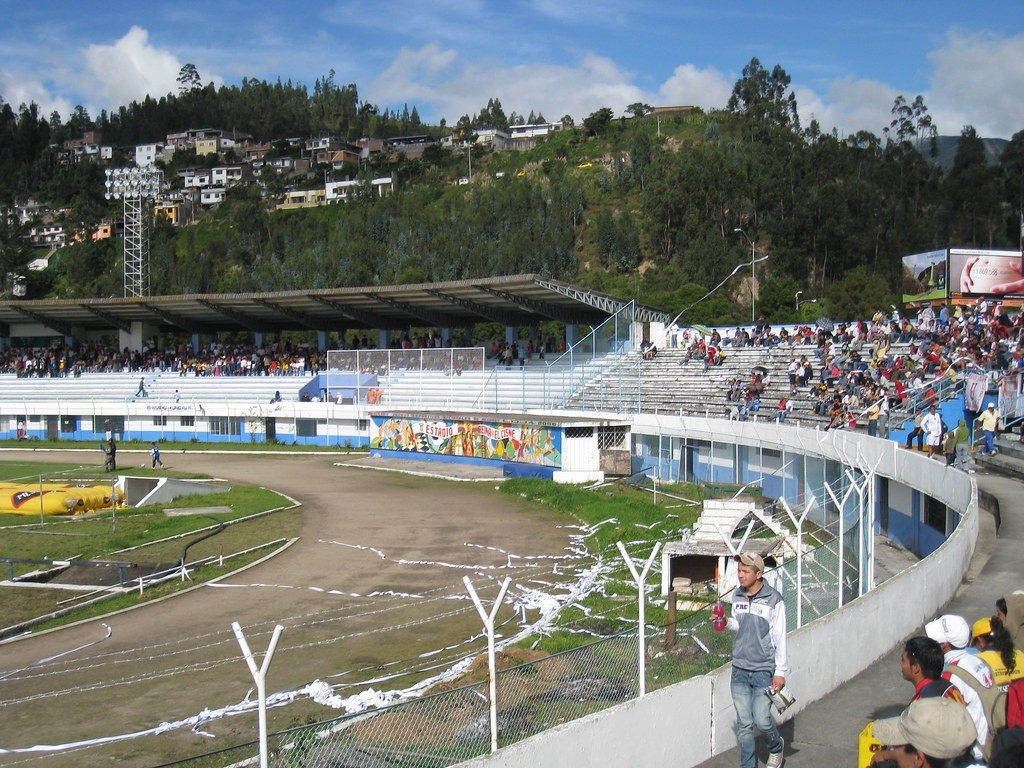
0,343 -> 1024,480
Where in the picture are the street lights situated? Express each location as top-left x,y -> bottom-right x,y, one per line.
733,227 -> 756,323
794,290 -> 817,311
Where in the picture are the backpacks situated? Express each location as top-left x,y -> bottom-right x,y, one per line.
944,655 -> 1008,764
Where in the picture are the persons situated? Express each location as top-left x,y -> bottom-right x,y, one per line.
135,377 -> 145,396
0,331 -> 565,377
301,392 -> 311,402
323,388 -> 333,402
150,441 -> 165,469
367,387 -> 383,405
270,390 -> 282,404
174,390 -> 180,403
311,393 -> 322,402
18,419 -> 24,441
707,551 -> 788,768
865,589 -> 1024,768
335,392 -> 343,405
105,438 -> 116,471
639,296 -> 1024,475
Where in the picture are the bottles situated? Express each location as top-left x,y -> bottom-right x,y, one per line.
714,599 -> 724,631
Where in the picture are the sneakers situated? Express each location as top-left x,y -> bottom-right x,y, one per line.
767,737 -> 785,768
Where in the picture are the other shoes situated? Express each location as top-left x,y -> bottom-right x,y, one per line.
991,450 -> 996,456
905,446 -> 913,450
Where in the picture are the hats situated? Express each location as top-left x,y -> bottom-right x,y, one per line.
988,403 -> 994,408
925,614 -> 970,648
872,696 -> 979,759
970,618 -> 992,647
696,296 -> 1018,430
734,552 -> 764,572
